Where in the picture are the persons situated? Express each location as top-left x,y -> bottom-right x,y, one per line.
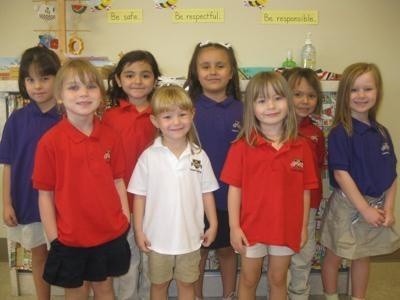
280,67 -> 327,299
103,49 -> 164,300
32,56 -> 131,299
219,71 -> 320,300
184,40 -> 245,300
0,45 -> 68,299
126,83 -> 222,299
316,61 -> 400,300
